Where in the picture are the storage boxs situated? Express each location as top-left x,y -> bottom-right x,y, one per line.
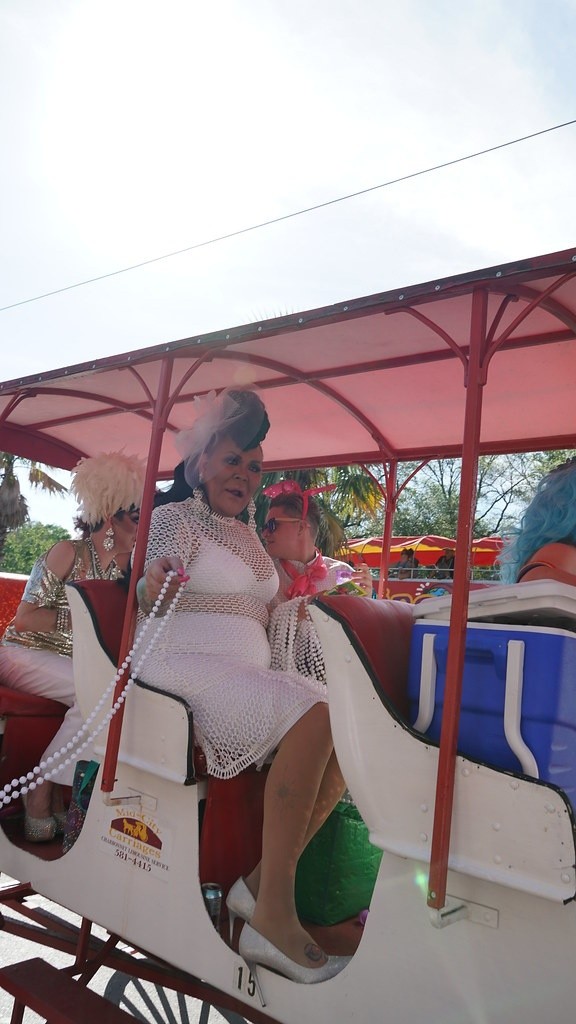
407,578 -> 576,819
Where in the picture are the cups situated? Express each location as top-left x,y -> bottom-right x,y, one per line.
335,570 -> 360,587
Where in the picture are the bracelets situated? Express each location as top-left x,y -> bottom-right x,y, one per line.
56,608 -> 69,634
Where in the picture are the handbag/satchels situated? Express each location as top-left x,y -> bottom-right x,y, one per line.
61,760 -> 98,855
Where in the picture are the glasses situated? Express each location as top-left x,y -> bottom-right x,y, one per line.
262,517 -> 311,534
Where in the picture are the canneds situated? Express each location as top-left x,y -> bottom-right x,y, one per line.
201,882 -> 222,934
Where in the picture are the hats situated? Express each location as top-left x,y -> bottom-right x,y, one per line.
443,547 -> 455,551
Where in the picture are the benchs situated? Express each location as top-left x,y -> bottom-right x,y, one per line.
0,570 -> 576,984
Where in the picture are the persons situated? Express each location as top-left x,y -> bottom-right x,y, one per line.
261,479 -> 372,679
0,451 -> 145,840
392,548 -> 419,580
497,458 -> 576,586
428,547 -> 455,579
130,382 -> 353,983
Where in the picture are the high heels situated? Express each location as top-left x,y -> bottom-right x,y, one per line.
225,876 -> 256,948
53,809 -> 67,834
21,778 -> 56,842
239,922 -> 353,1008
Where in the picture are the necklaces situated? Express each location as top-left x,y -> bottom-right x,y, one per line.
266,594 -> 327,684
84,538 -> 113,580
0,571 -> 185,808
